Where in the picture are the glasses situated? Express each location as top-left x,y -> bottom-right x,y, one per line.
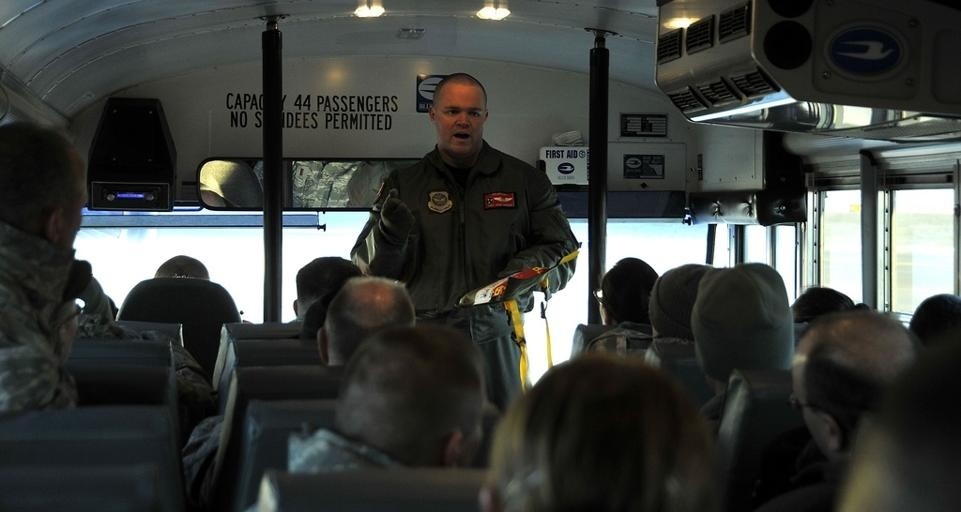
592,289 -> 605,308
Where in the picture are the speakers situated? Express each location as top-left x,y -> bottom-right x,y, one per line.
86,94 -> 175,213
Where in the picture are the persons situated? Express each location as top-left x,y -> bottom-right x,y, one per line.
1,257 -> 961,512
54,181 -> 120,325
1,122 -> 85,410
350,74 -> 584,391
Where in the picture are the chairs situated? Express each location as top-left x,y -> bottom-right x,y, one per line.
1,277 -> 826,511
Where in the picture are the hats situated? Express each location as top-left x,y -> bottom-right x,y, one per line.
692,263 -> 794,382
649,262 -> 721,335
598,258 -> 654,325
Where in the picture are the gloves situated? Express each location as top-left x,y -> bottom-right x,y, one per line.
378,189 -> 416,240
